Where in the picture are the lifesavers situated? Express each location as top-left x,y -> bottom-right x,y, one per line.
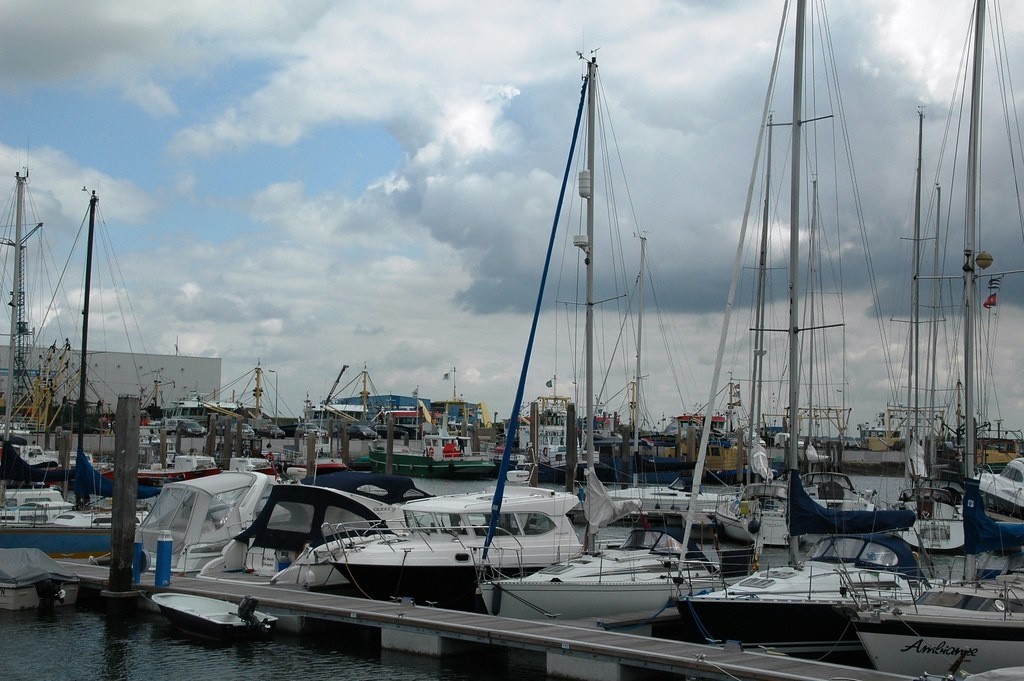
543,446 -> 547,455
429,446 -> 434,457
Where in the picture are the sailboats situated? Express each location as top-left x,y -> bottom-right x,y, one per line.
477,0 -> 1023,675
0,165 -> 148,528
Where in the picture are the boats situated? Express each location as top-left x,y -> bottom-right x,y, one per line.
283,435 -> 347,476
138,453 -> 276,482
368,410 -> 497,480
135,472 -> 584,613
151,592 -> 279,639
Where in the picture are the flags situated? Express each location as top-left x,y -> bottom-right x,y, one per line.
987,276 -> 999,288
983,292 -> 996,308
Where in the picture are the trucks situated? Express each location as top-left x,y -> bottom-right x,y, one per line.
160,418 -> 190,435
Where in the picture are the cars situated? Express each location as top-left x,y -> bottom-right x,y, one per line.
347,425 -> 377,441
176,420 -> 207,437
296,423 -> 321,437
266,426 -> 285,439
231,423 -> 256,438
